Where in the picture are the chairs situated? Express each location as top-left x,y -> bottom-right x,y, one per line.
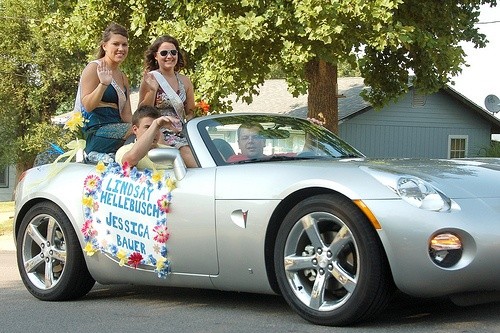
212,138 -> 236,162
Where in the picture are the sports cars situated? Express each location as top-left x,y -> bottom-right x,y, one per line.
14,113 -> 500,324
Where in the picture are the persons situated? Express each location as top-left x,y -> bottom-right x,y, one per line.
138,35 -> 198,168
73,23 -> 137,164
114,105 -> 184,170
225,123 -> 315,163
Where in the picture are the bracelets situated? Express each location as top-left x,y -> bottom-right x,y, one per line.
101,82 -> 109,87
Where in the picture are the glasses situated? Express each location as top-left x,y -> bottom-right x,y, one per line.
155,48 -> 179,57
